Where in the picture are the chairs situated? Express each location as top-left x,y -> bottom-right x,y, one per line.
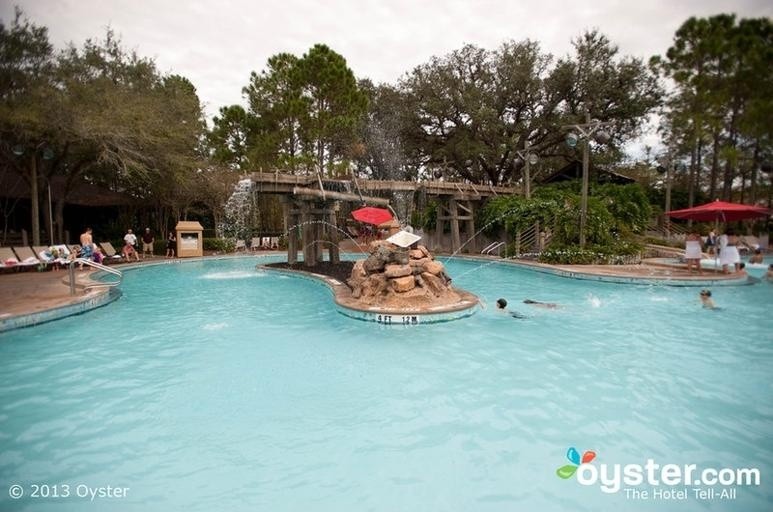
233,236 -> 280,252
684,233 -> 764,255
0,240 -> 131,273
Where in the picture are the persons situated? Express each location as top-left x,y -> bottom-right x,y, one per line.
682,228 -> 773,277
124,229 -> 138,249
701,290 -> 713,307
79,228 -> 95,271
524,299 -> 566,309
59,246 -> 103,266
141,228 -> 155,258
476,298 -> 528,318
122,243 -> 140,263
165,232 -> 176,258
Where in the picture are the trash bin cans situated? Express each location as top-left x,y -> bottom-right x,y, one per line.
175,221 -> 204,257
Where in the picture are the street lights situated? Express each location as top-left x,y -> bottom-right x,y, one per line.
12,141 -> 58,246
560,110 -> 616,251
520,138 -> 539,196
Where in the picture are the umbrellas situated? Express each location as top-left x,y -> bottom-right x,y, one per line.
664,199 -> 770,274
